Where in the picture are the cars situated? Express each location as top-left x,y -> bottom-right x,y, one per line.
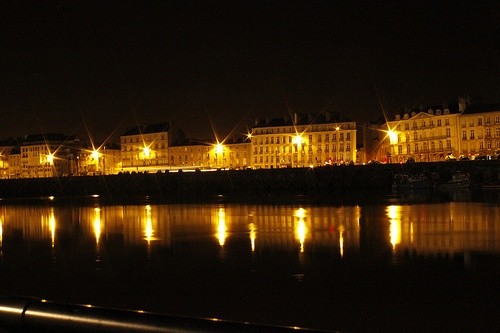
445,153 -> 500,160
368,159 -> 377,165
327,159 -> 354,166
406,158 -> 415,163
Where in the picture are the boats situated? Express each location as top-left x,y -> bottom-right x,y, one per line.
393,170 -> 481,189
